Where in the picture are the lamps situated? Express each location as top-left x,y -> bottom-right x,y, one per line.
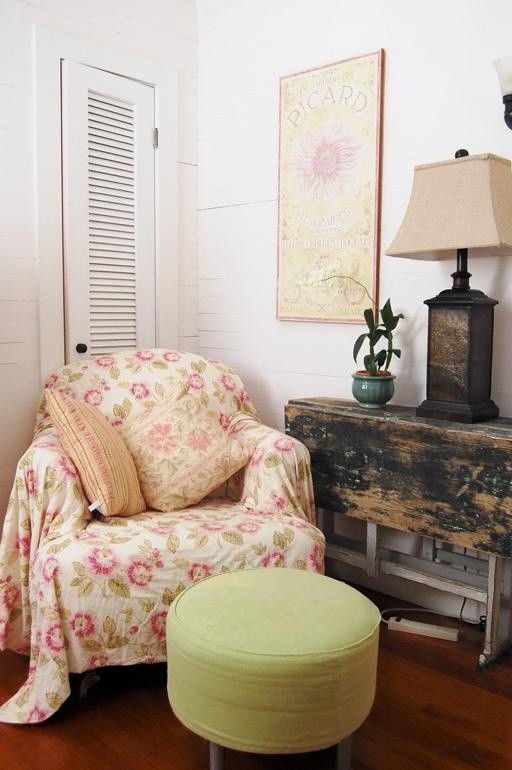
383,147 -> 512,427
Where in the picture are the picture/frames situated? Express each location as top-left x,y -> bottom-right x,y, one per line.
276,47 -> 388,326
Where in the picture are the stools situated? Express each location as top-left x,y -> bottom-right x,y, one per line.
165,567 -> 384,769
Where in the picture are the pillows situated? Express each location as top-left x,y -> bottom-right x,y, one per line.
43,386 -> 251,518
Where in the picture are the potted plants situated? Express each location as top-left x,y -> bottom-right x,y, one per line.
319,272 -> 406,410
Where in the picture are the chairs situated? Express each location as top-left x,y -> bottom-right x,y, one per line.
15,347 -> 330,709
